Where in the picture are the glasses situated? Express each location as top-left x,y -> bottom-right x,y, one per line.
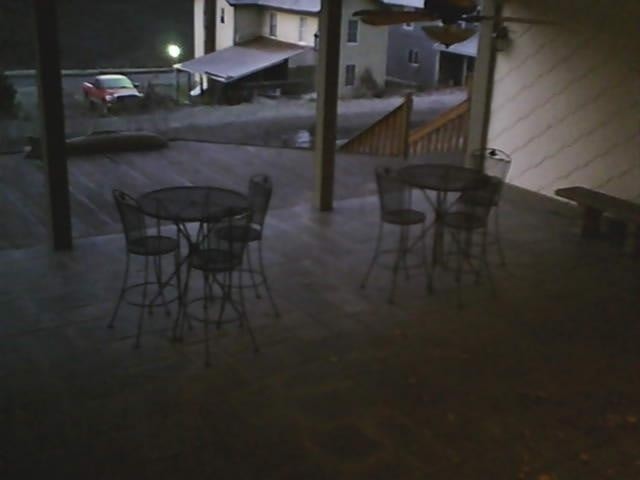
419,21 -> 477,49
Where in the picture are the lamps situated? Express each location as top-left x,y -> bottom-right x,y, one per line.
106,172 -> 281,370
357,146 -> 513,310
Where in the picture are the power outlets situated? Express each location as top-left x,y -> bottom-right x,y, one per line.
82,73 -> 146,116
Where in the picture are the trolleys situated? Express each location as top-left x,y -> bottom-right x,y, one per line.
352,0 -> 565,25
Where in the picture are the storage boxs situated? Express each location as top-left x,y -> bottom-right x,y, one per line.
555,186 -> 640,249
